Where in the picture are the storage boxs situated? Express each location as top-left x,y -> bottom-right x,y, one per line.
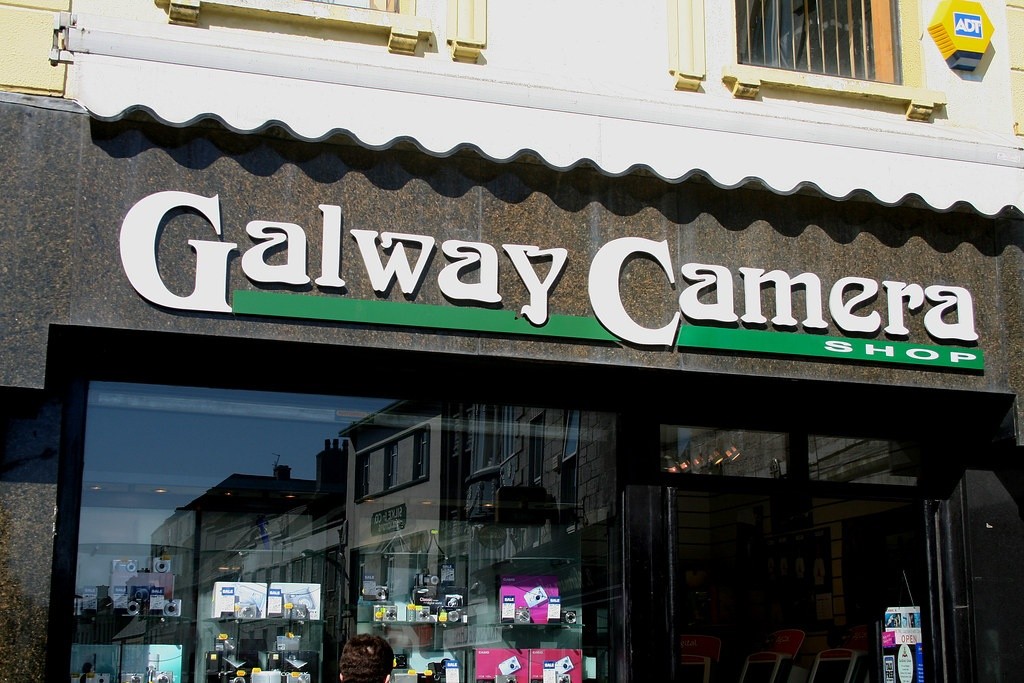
529,647 -> 583,683
212,582 -> 267,620
269,583 -> 321,620
495,575 -> 562,623
472,648 -> 529,683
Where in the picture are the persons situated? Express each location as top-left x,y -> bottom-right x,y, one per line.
340,633 -> 394,683
675,561 -> 710,634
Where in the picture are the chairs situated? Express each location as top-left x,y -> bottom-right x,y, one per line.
674,624 -> 870,683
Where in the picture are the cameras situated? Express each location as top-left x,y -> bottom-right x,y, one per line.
555,656 -> 574,674
70,665 -> 173,683
557,673 -> 571,683
561,610 -> 576,624
494,674 -> 516,683
513,607 -> 530,624
498,656 -> 521,676
73,556 -> 181,617
363,574 -> 467,683
204,602 -> 312,683
523,585 -> 548,608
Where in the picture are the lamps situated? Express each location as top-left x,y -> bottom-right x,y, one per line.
667,447 -> 739,473
